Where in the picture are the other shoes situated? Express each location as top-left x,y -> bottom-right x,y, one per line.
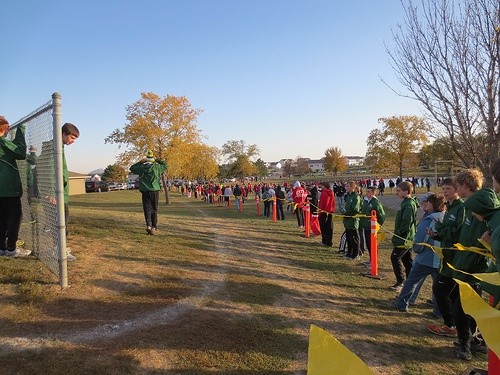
146,226 -> 156,234
464,339 -> 488,354
0,249 -> 7,256
386,301 -> 407,311
5,248 -> 32,257
388,283 -> 403,291
53,248 -> 77,261
455,346 -> 472,361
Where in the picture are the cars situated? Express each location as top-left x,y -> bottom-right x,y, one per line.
85,180 -> 139,193
224,176 -> 236,182
159,177 -> 214,187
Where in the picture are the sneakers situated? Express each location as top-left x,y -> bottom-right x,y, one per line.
428,322 -> 458,338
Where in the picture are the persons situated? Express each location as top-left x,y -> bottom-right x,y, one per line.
467,158 -> 500,375
333,181 -> 348,208
253,180 -> 321,236
317,182 -> 335,247
387,192 -> 447,319
361,186 -> 386,268
427,170 -> 487,360
388,181 -> 418,291
130,150 -> 169,235
182,177 -> 251,210
389,177 -> 400,193
0,115 -> 49,256
338,181 -> 365,261
37,123 -> 80,262
358,177 -> 385,196
406,176 -> 430,194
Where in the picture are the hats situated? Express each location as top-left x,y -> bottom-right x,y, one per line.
294,181 -> 300,187
418,191 -> 434,201
146,150 -> 154,162
322,181 -> 331,189
